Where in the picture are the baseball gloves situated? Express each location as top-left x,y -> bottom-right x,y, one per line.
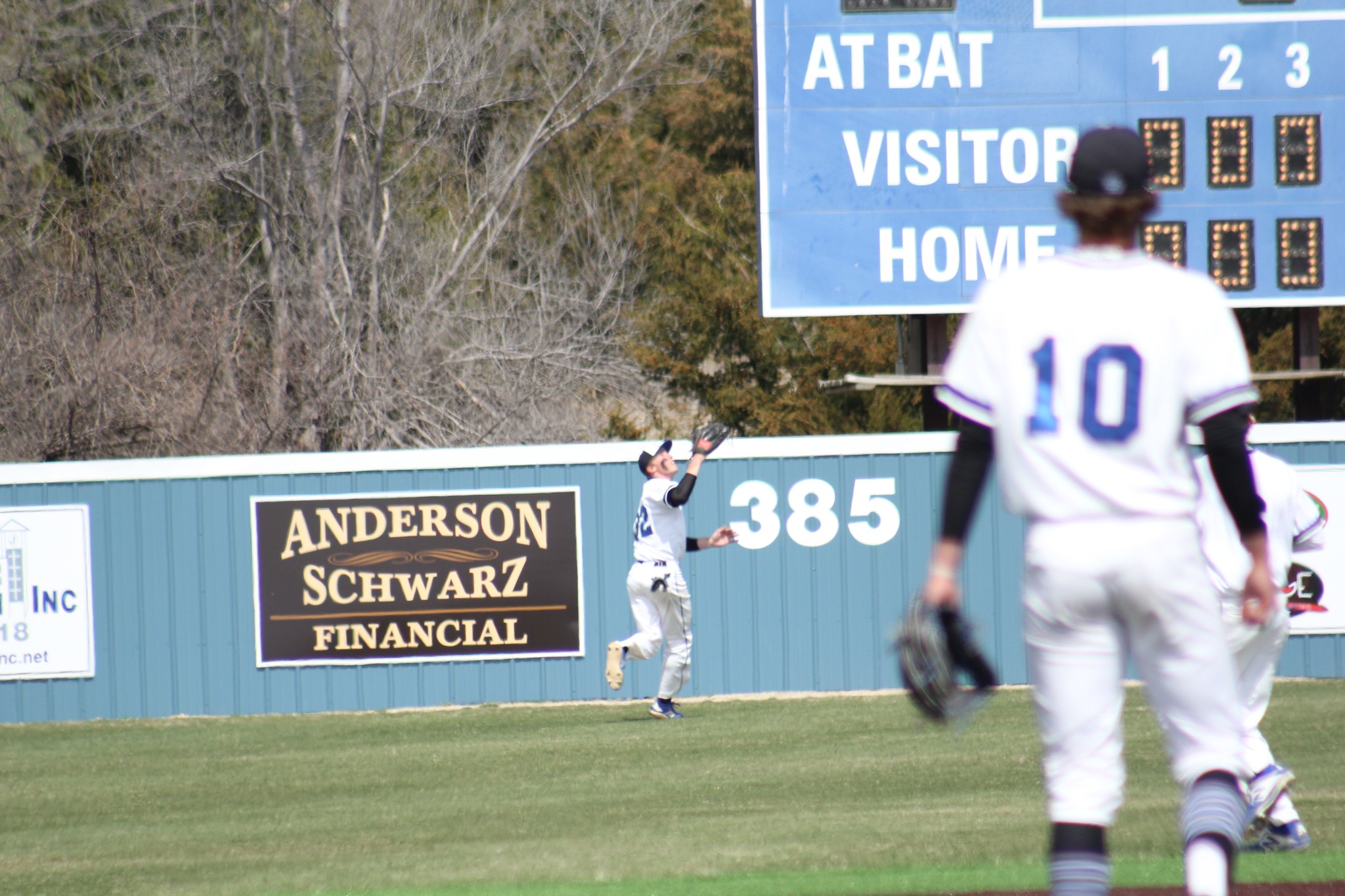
891,596 -> 1001,728
690,420 -> 733,457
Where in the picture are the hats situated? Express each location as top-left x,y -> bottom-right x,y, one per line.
638,440 -> 672,474
1070,127 -> 1148,194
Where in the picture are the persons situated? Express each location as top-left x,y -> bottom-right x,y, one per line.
605,420 -> 740,718
898,125 -> 1275,896
1190,413 -> 1313,853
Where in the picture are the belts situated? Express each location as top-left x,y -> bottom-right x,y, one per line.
637,560 -> 667,566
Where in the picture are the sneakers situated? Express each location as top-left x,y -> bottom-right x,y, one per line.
1246,820 -> 1312,853
1247,765 -> 1295,821
606,641 -> 626,690
649,699 -> 684,719
